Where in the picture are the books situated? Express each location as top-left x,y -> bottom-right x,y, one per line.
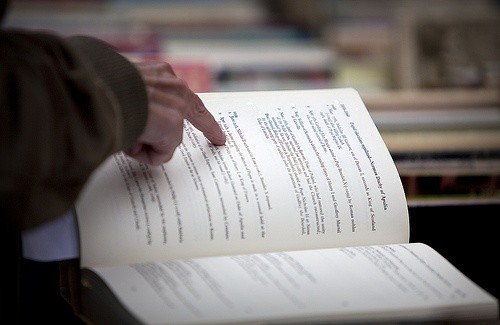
72,87 -> 498,325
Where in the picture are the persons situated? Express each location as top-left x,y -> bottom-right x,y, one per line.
1,23 -> 227,238
